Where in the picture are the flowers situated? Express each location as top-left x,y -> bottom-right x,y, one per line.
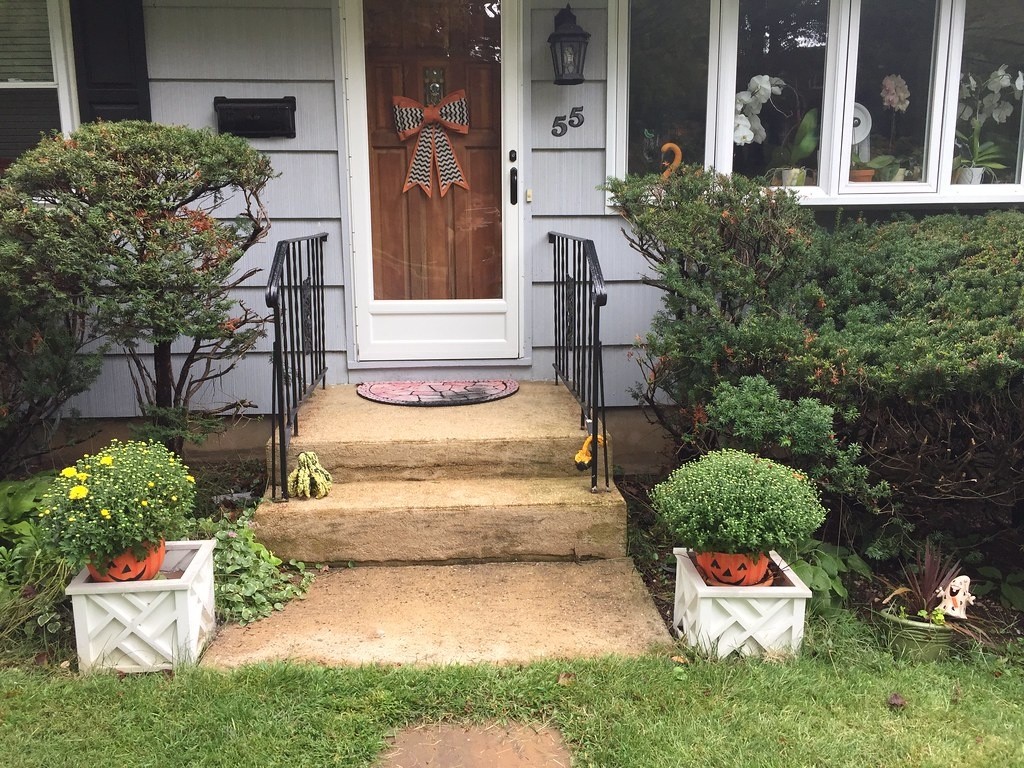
733,75 -> 807,167
953,63 -> 1023,171
42,438 -> 194,572
645,449 -> 829,555
880,74 -> 911,166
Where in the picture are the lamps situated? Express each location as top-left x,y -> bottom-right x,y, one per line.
547,3 -> 591,85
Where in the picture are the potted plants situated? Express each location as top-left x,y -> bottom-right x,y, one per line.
882,534 -> 964,666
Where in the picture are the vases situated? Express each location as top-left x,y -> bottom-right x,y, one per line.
88,533 -> 165,582
696,550 -> 770,585
883,167 -> 905,181
956,167 -> 984,184
782,168 -> 806,186
850,169 -> 875,182
